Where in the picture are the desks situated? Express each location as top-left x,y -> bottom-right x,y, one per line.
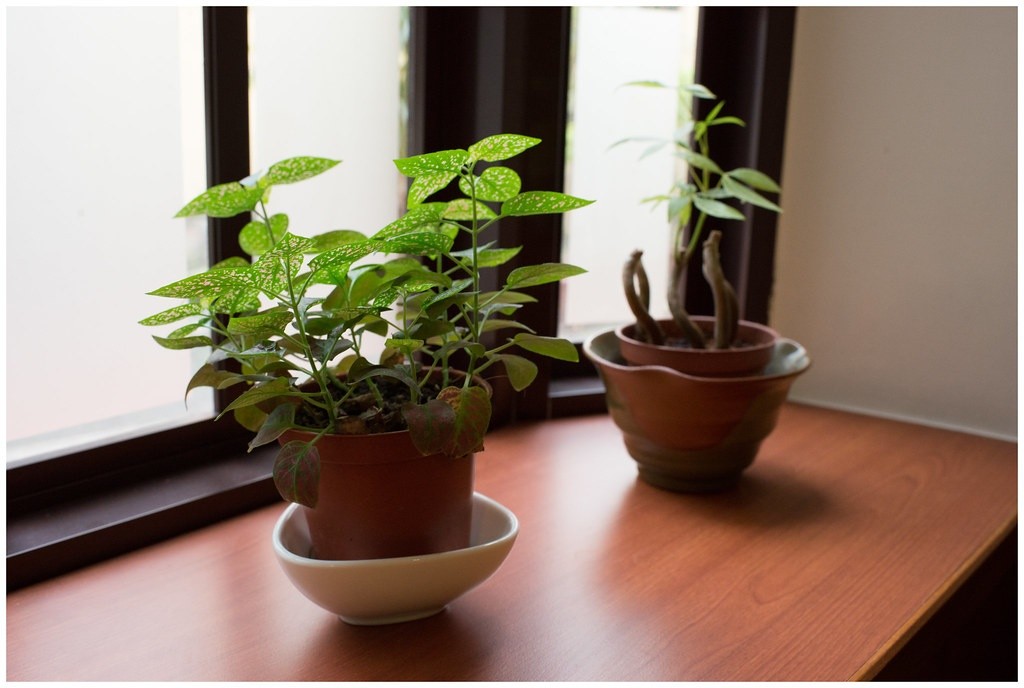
6,384 -> 1017,682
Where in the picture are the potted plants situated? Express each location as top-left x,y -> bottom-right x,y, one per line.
586,64 -> 813,496
138,134 -> 598,626
